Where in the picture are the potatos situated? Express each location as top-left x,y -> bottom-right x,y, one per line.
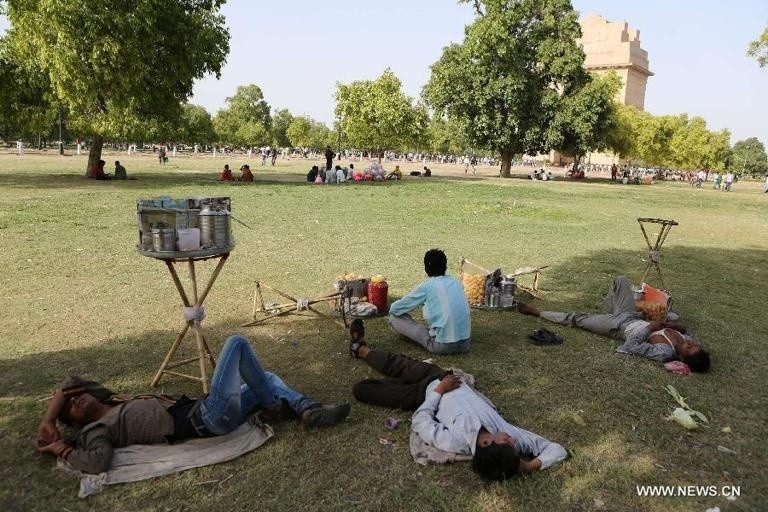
461,273 -> 485,306
635,301 -> 668,321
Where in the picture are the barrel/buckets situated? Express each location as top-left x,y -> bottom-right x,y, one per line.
337,276 -> 372,300
488,290 -> 501,306
136,205 -> 176,250
197,203 -> 236,249
175,207 -> 201,241
499,275 -> 515,296
632,290 -> 645,300
152,228 -> 175,252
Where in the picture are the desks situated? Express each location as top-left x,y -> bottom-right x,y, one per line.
134,239 -> 236,396
634,218 -> 679,292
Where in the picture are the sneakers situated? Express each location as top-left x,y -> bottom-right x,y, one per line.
303,404 -> 351,426
349,318 -> 367,358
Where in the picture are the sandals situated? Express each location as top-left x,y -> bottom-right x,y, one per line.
528,328 -> 564,346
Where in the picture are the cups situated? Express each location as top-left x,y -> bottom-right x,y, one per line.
478,294 -> 514,308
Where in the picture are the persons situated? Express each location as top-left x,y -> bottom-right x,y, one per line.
530,169 -> 555,180
349,319 -> 567,481
611,164 -> 630,184
325,146 -> 336,170
114,161 -> 126,180
696,168 -> 704,188
463,156 -> 477,175
388,250 -> 471,355
159,147 -> 166,164
34,336 -> 350,475
220,146 -> 277,181
715,171 -> 738,191
763,175 -> 768,193
92,160 -> 108,179
422,167 -> 431,176
307,164 -> 402,183
516,275 -> 710,373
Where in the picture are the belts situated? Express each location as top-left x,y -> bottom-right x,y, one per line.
187,392 -> 215,436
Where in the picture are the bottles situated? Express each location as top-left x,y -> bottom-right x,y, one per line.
367,276 -> 389,315
